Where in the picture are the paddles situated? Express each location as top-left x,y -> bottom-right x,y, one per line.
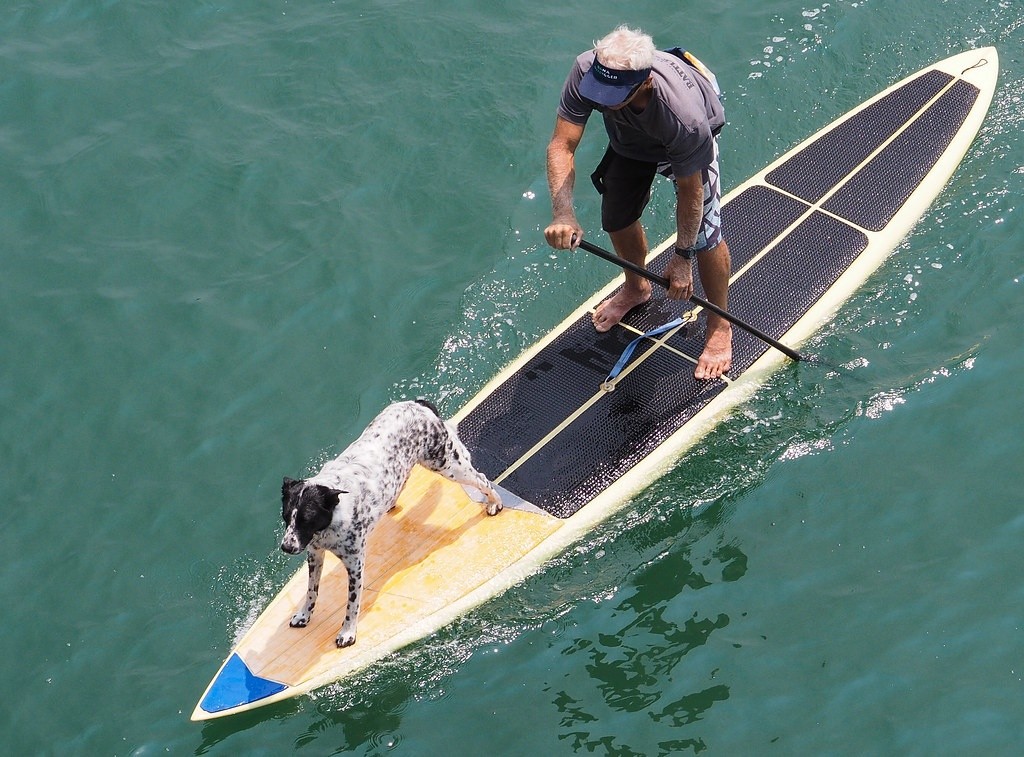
569,231 -> 808,366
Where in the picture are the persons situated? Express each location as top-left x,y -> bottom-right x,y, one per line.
544,23 -> 734,380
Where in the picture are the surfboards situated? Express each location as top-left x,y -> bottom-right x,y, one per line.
188,44 -> 1005,723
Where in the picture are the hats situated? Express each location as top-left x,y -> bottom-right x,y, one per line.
578,53 -> 651,106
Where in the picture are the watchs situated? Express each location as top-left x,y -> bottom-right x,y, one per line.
673,246 -> 697,259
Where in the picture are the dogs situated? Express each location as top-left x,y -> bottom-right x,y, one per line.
280,400 -> 501,648
